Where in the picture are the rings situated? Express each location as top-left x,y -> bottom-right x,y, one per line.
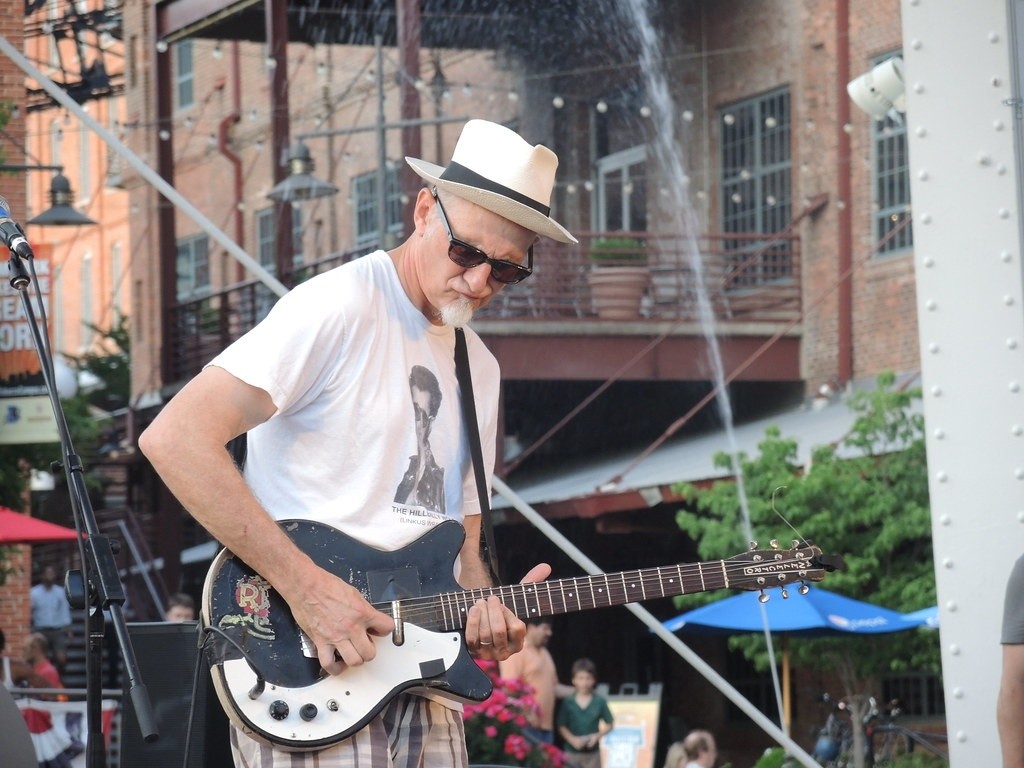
480,641 -> 494,645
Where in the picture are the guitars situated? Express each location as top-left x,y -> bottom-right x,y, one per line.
198,516 -> 844,755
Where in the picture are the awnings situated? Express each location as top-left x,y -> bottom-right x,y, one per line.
470,367 -> 923,535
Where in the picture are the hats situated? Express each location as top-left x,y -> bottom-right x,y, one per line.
405,119 -> 579,244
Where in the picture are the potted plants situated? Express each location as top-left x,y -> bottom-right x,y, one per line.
587,239 -> 652,319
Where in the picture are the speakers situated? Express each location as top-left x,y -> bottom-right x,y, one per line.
117,620 -> 236,768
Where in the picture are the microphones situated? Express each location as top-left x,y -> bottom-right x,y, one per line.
0,195 -> 33,259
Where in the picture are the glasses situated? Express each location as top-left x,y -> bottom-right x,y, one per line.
432,185 -> 533,285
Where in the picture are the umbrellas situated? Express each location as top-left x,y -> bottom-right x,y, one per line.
649,583 -> 924,757
903,602 -> 940,630
0,505 -> 87,543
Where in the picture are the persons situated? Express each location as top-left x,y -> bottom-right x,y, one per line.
498,619 -> 576,747
395,366 -> 446,513
994,552 -> 1024,768
555,658 -> 614,768
135,118 -> 552,768
31,564 -> 73,654
162,592 -> 193,622
663,731 -> 715,768
0,629 -> 66,768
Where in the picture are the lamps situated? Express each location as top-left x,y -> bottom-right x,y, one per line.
846,57 -> 906,118
